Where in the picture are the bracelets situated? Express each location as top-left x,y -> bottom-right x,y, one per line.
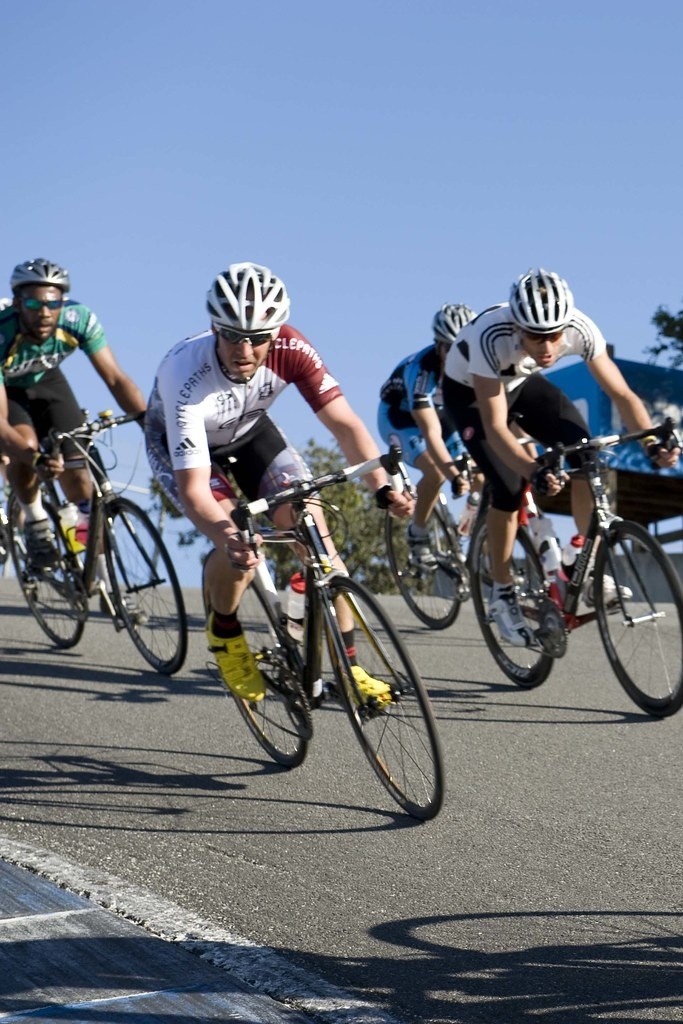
640,435 -> 657,447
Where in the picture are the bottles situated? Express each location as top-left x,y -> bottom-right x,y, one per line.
528,512 -> 560,571
286,570 -> 307,644
467,491 -> 480,515
557,535 -> 585,582
57,499 -> 87,555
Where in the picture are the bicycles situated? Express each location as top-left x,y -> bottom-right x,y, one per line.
378,449 -> 539,631
4,408 -> 192,678
181,435 -> 450,822
465,412 -> 682,722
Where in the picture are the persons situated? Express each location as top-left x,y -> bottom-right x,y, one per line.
0,258 -> 148,573
377,304 -> 487,571
143,262 -> 415,709
440,267 -> 683,647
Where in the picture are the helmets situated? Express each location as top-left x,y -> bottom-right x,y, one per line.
10,257 -> 71,295
510,268 -> 574,334
206,262 -> 290,337
433,303 -> 478,346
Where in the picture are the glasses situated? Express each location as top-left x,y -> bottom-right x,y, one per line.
16,297 -> 64,310
519,329 -> 565,342
214,325 -> 273,347
441,344 -> 453,351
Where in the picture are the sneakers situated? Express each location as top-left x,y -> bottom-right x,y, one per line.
488,592 -> 535,647
406,524 -> 439,570
342,663 -> 392,708
205,611 -> 264,701
560,553 -> 632,607
24,518 -> 61,572
99,589 -> 149,624
